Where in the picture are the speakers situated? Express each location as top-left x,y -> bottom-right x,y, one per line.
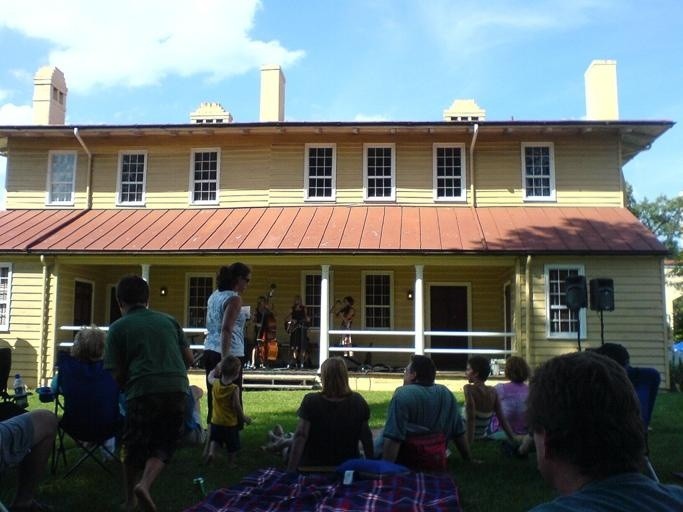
565,275 -> 587,313
589,278 -> 615,312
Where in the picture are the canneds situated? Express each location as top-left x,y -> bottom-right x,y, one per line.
193,478 -> 206,496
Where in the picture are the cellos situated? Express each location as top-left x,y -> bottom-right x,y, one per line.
259,283 -> 278,362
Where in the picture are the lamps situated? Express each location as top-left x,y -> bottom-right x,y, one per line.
159,286 -> 167,295
406,288 -> 414,301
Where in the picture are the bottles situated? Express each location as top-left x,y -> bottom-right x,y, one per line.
12,372 -> 27,407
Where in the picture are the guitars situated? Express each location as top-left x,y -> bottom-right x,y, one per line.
284,313 -> 319,335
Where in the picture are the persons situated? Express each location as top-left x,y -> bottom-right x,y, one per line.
50,272 -> 204,512
265,356 -> 375,475
201,261 -> 253,458
248,295 -> 269,371
358,355 -> 485,466
207,353 -> 251,469
515,340 -> 631,459
1,409 -> 59,512
460,356 -> 514,444
490,355 -> 531,435
525,349 -> 683,512
334,295 -> 356,357
286,295 -> 308,368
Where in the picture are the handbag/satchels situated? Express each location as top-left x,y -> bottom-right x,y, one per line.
404,431 -> 447,472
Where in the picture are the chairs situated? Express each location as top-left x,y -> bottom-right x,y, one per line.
628,362 -> 661,440
0,348 -> 32,421
49,355 -> 122,497
